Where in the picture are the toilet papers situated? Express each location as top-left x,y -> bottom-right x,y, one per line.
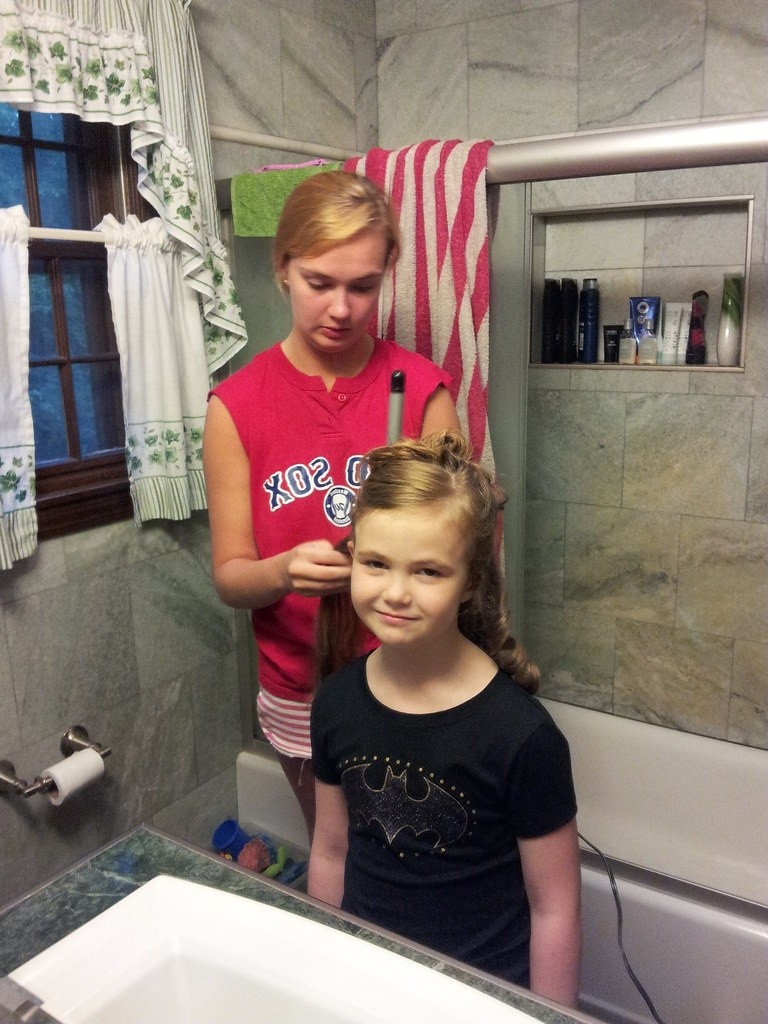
41,747 -> 105,807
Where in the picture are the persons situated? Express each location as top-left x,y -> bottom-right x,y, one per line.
208,170 -> 463,848
306,433 -> 583,1010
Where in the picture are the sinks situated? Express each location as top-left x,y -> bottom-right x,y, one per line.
6,877 -> 541,1024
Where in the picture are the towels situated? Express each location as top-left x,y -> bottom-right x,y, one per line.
340,139 -> 506,583
231,161 -> 340,238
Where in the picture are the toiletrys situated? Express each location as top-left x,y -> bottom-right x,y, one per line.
540,273 -> 742,368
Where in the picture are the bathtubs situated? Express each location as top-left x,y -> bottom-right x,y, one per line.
234,691 -> 768,1024
